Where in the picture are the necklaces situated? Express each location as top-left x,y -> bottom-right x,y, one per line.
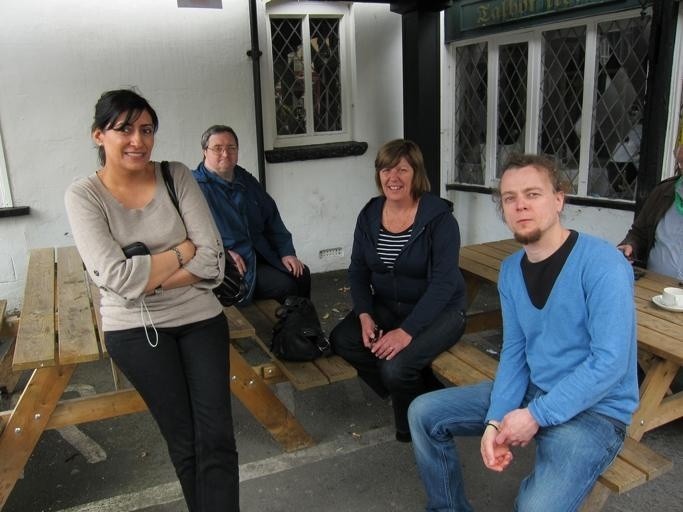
384,209 -> 412,232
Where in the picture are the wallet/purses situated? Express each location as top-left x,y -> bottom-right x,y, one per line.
122,241 -> 151,258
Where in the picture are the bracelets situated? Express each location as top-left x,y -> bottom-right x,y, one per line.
169,246 -> 183,270
486,422 -> 497,431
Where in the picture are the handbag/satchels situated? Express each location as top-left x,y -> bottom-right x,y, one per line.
270,295 -> 331,362
213,245 -> 249,307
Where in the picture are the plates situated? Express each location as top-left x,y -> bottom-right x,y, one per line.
652,294 -> 681,313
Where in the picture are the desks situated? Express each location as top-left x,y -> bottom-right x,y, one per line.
456,237 -> 682,512
0,244 -> 312,512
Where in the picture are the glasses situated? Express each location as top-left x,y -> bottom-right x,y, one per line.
208,145 -> 238,154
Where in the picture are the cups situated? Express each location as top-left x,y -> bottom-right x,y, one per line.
662,286 -> 681,306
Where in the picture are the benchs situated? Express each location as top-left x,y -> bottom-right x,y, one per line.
238,297 -> 358,394
432,342 -> 674,511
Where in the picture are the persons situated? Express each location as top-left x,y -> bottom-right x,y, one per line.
613,129 -> 683,291
189,125 -> 311,304
404,153 -> 641,511
61,88 -> 242,512
328,140 -> 472,442
